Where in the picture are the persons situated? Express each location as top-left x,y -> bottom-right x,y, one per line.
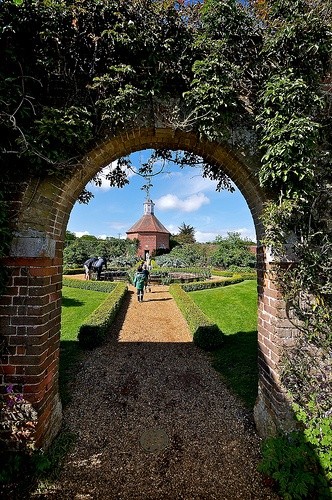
84,256 -> 102,280
134,267 -> 147,302
142,262 -> 151,290
93,256 -> 108,280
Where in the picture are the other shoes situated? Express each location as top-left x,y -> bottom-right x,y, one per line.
138,300 -> 143,302
